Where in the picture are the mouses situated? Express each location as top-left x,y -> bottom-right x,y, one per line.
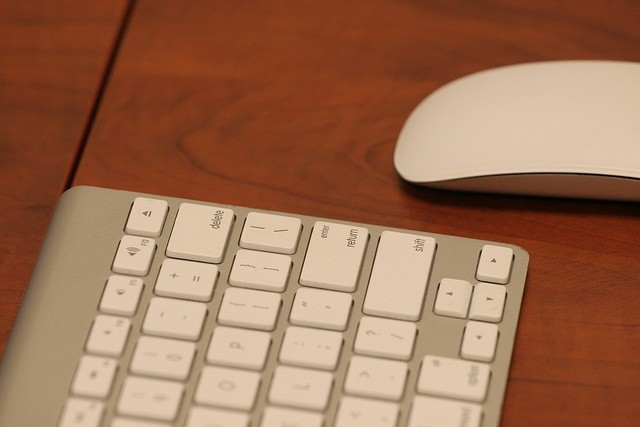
393,60 -> 639,201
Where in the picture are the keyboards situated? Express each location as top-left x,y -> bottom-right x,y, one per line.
1,185 -> 530,427
